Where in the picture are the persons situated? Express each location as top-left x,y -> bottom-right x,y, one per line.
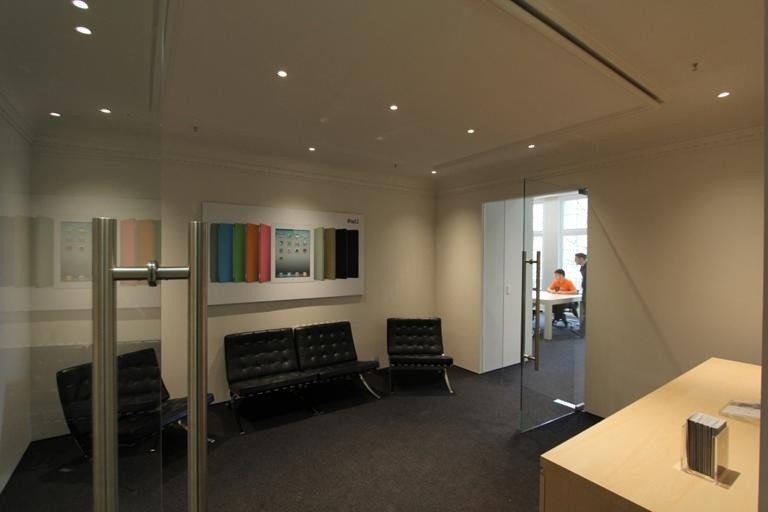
572,253 -> 586,336
546,269 -> 577,328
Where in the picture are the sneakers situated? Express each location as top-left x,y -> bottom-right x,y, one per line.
553,320 -> 565,327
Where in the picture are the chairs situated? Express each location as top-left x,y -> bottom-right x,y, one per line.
386,318 -> 453,394
294,320 -> 382,415
57,347 -> 216,464
224,328 -> 320,436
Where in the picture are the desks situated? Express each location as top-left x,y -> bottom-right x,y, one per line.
531,290 -> 582,340
539,356 -> 768,512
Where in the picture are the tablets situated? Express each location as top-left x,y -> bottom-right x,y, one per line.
271,225 -> 315,283
53,217 -> 93,289
